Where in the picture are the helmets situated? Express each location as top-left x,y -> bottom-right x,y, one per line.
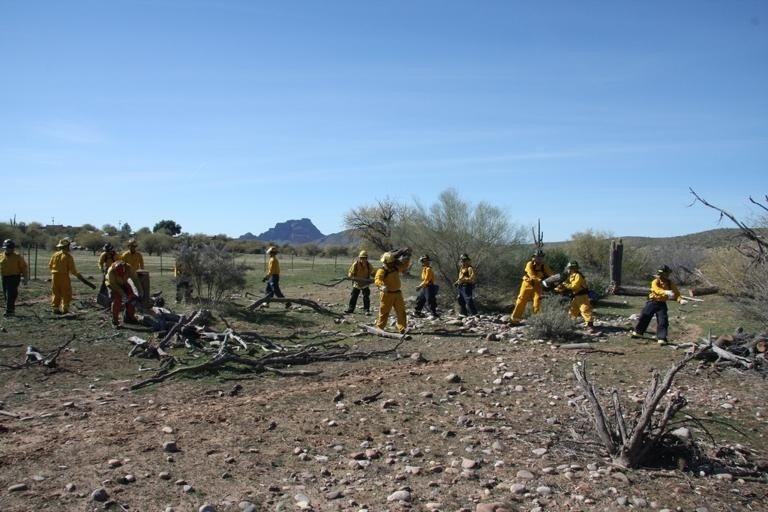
380,251 -> 395,264
359,250 -> 369,258
2,239 -> 20,249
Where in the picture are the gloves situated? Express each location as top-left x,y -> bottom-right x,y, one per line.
136,289 -> 144,298
679,299 -> 689,305
454,279 -> 463,289
22,278 -> 29,285
665,290 -> 675,299
415,284 -> 423,292
381,285 -> 390,293
262,275 -> 270,283
121,293 -> 128,307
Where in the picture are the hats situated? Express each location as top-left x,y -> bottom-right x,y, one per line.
458,253 -> 473,261
112,261 -> 128,276
419,254 -> 433,262
56,239 -> 70,247
531,249 -> 546,257
266,247 -> 281,254
656,264 -> 673,275
127,238 -> 139,247
564,260 -> 582,270
102,242 -> 115,252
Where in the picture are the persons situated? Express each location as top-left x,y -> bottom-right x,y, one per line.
343,249 -> 374,315
413,253 -> 439,320
105,260 -> 145,327
97,242 -> 119,272
119,237 -> 145,273
508,250 -> 557,328
1,238 -> 32,319
451,251 -> 480,318
259,245 -> 293,310
552,260 -> 594,331
47,239 -> 80,314
173,251 -> 198,302
631,263 -> 690,347
374,250 -> 411,334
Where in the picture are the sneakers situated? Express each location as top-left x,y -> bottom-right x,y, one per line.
4,309 -> 16,316
123,315 -> 139,324
630,331 -> 643,339
584,321 -> 594,328
657,338 -> 669,345
509,320 -> 520,327
63,308 -> 72,314
111,318 -> 120,326
52,308 -> 61,314
